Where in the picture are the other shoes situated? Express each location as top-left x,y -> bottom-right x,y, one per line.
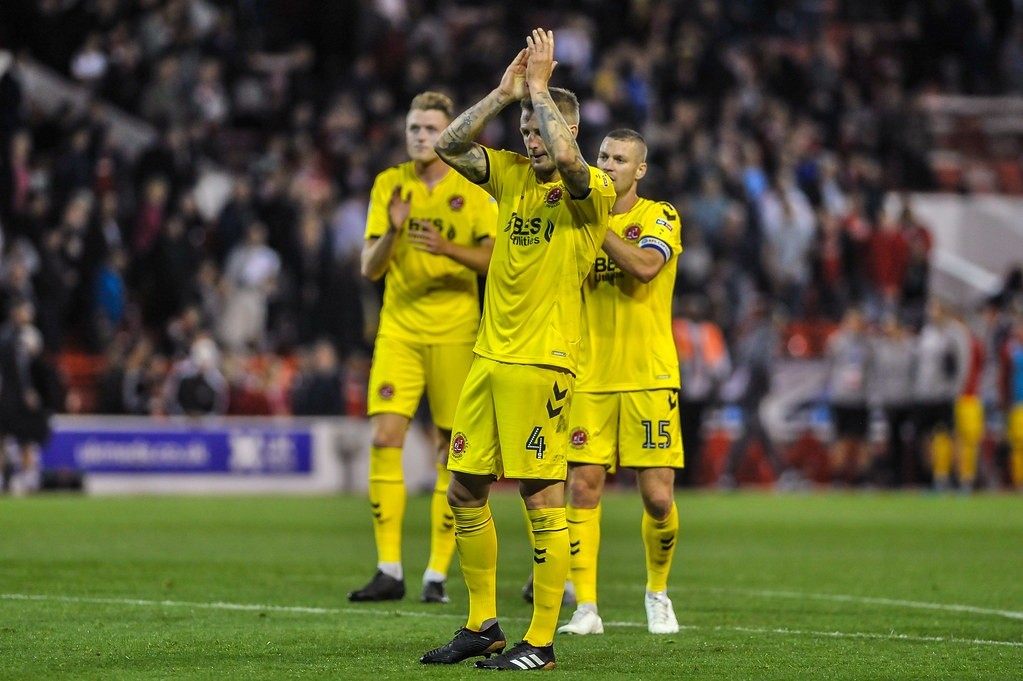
777,472 -> 798,492
719,476 -> 741,496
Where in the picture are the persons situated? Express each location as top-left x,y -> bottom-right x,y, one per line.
556,127 -> 684,636
2,0 -> 1023,493
345,91 -> 501,605
419,26 -> 617,673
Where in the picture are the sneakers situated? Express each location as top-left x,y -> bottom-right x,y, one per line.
557,604 -> 604,635
521,576 -> 576,606
346,570 -> 405,602
474,641 -> 556,671
421,581 -> 449,603
419,621 -> 507,663
645,591 -> 679,634
